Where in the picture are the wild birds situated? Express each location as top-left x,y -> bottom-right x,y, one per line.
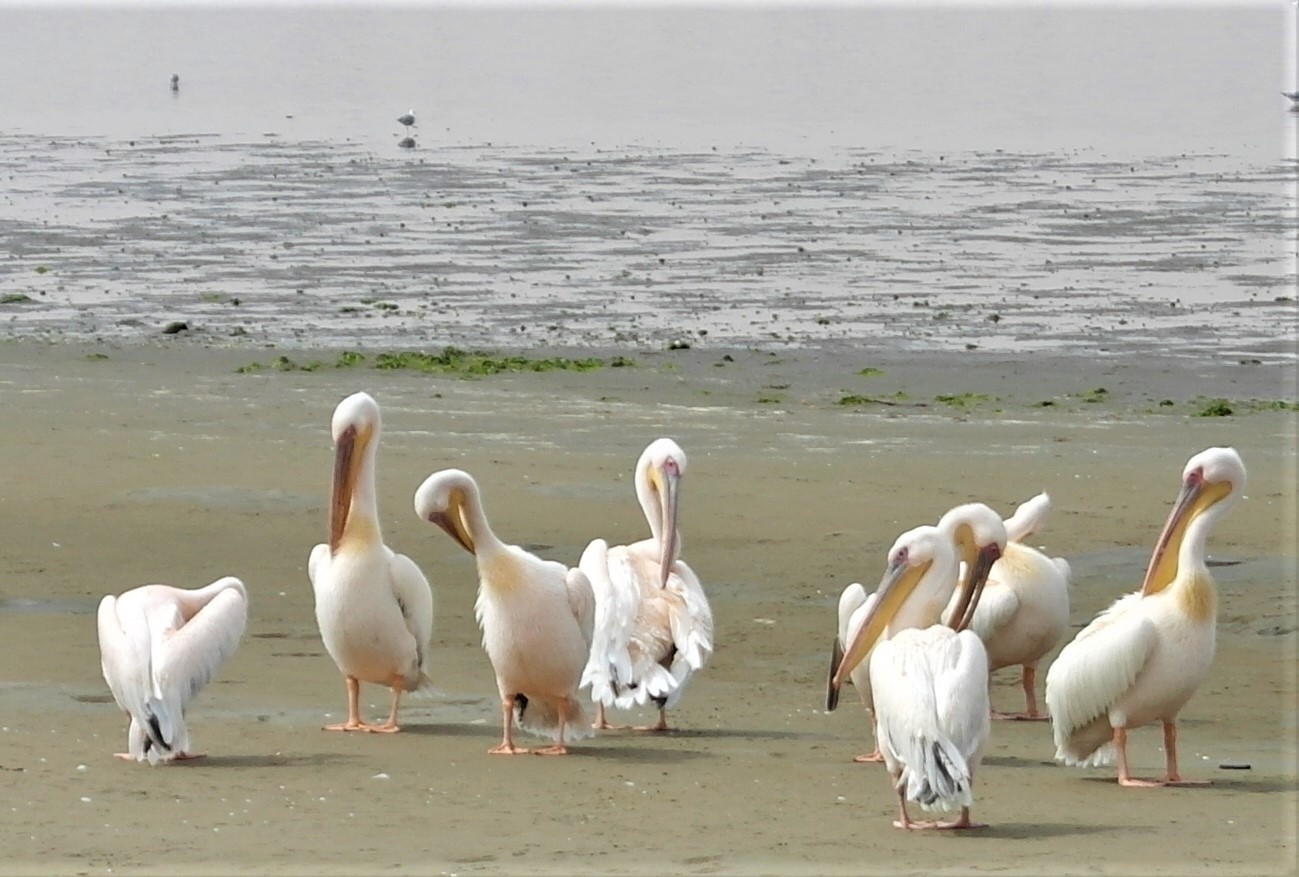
581,439 -> 715,734
824,491 -> 1075,830
307,391 -> 433,737
413,465 -> 596,757
397,108 -> 417,133
96,575 -> 249,764
1047,446 -> 1248,790
